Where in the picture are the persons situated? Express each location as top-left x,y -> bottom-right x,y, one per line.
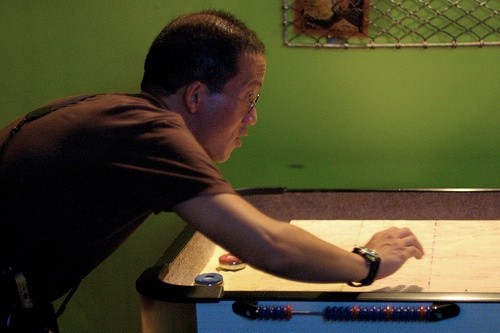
2,9 -> 424,333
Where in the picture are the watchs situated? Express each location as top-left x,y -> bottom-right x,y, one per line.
347,245 -> 384,287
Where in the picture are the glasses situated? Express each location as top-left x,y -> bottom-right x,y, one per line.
209,86 -> 261,112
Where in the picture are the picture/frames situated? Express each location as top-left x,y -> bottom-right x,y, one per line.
293,0 -> 370,38
